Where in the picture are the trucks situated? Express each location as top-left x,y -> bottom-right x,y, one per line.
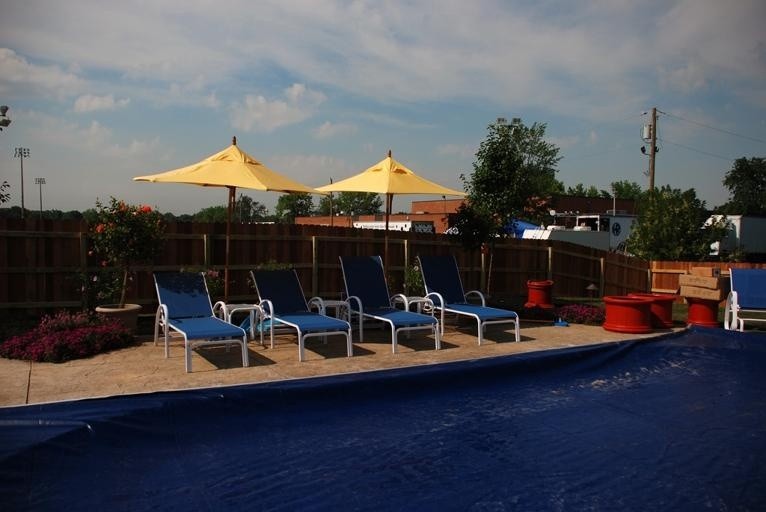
695,212 -> 766,262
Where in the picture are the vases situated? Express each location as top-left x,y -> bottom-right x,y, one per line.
92,303 -> 140,333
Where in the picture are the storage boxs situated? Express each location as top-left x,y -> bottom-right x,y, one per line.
677,264 -> 729,303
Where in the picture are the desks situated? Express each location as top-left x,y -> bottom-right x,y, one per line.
391,297 -> 434,337
310,299 -> 351,345
221,303 -> 264,352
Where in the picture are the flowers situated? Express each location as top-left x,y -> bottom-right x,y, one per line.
86,196 -> 167,307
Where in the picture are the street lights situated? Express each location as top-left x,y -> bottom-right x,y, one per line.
441,195 -> 447,212
641,107 -> 657,191
33,177 -> 45,217
0,102 -> 12,133
13,147 -> 30,220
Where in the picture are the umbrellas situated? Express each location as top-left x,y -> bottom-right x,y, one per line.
133,135 -> 325,308
316,149 -> 471,297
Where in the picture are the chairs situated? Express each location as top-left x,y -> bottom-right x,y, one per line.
151,269 -> 249,373
415,252 -> 521,346
249,268 -> 354,363
722,266 -> 765,335
338,253 -> 442,354
